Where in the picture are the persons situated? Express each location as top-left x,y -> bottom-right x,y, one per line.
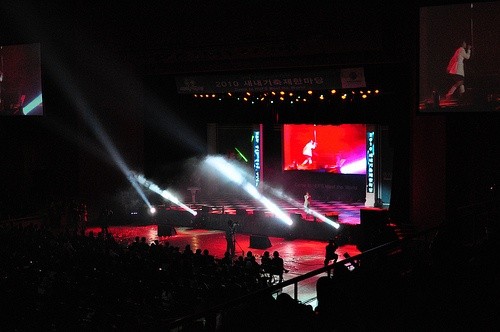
1,202 -> 500,331
298,139 -> 317,170
303,191 -> 312,218
444,38 -> 471,100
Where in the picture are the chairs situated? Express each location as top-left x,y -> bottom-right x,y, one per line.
245,261 -> 285,289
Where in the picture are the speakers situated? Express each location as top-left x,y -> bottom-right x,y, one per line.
360,207 -> 388,225
249,234 -> 271,250
157,223 -> 176,237
236,208 -> 247,215
253,210 -> 265,216
